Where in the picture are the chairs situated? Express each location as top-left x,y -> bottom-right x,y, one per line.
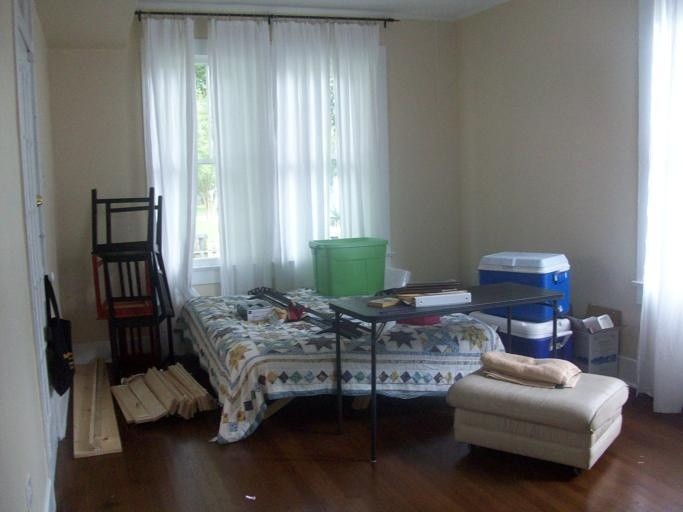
91,187 -> 175,377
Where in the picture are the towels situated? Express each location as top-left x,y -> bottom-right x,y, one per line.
481,349 -> 582,388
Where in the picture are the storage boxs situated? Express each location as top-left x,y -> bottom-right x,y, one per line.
477,251 -> 572,323
568,303 -> 622,377
308,237 -> 388,297
469,311 -> 575,362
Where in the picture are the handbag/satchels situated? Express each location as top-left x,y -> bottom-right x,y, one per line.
42,273 -> 76,398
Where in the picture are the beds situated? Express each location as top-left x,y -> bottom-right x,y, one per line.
182,289 -> 497,437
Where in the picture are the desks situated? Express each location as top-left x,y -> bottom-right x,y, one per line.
329,282 -> 564,462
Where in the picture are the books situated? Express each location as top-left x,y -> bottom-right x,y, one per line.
368,279 -> 472,309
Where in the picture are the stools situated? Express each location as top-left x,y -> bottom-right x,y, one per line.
445,364 -> 630,472
